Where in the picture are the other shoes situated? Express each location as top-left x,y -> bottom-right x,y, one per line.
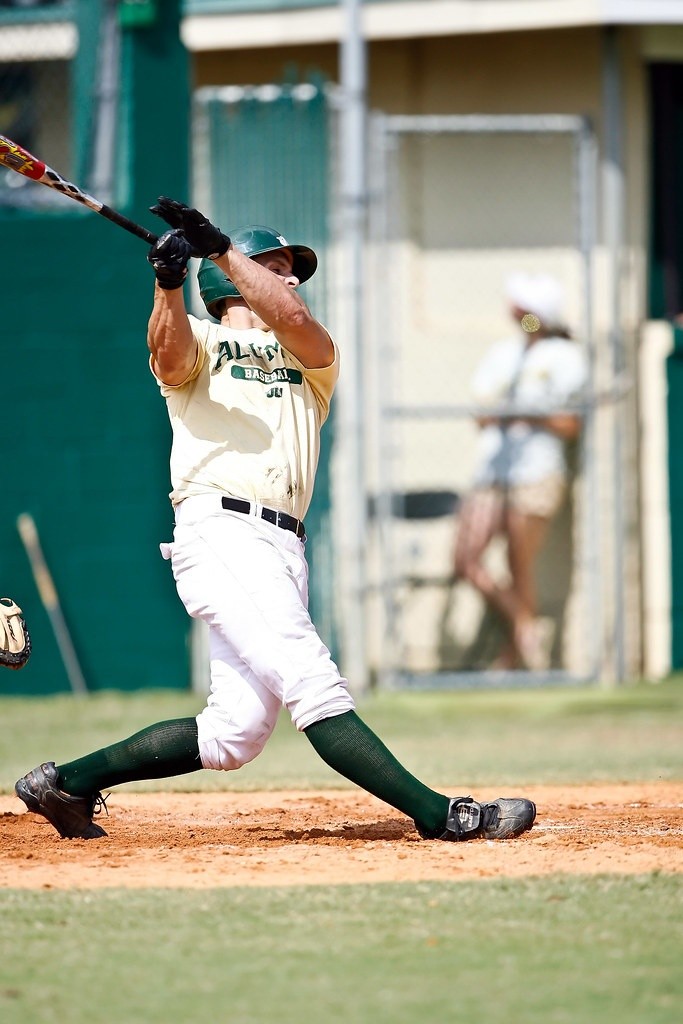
528,614 -> 555,673
484,659 -> 521,684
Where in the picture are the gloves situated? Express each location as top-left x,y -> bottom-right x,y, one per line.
149,196 -> 231,260
147,230 -> 193,290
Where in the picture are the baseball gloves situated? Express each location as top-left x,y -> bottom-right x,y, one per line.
0,596 -> 32,669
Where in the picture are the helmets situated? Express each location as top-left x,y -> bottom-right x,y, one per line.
197,224 -> 318,321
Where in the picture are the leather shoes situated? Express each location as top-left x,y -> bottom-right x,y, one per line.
222,496 -> 305,541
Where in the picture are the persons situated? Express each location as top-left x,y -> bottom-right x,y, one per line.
15,196 -> 537,843
451,268 -> 590,673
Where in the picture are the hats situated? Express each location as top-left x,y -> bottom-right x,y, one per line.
506,273 -> 567,320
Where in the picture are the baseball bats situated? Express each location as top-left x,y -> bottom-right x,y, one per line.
16,512 -> 88,693
0,136 -> 157,243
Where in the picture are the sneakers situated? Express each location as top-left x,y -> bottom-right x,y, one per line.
415,795 -> 536,841
15,762 -> 111,840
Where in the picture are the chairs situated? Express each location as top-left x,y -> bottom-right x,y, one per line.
360,491 -> 464,672
